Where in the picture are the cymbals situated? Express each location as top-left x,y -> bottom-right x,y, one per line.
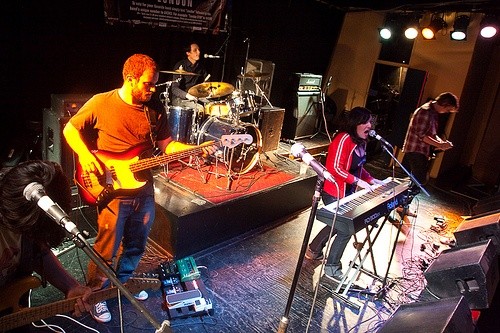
188,82 -> 234,98
238,73 -> 270,78
159,70 -> 199,75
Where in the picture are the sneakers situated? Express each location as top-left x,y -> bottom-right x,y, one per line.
132,289 -> 148,301
88,299 -> 111,323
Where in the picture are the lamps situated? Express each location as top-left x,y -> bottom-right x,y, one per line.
377,9 -> 500,41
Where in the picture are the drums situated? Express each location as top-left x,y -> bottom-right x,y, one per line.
226,89 -> 258,117
202,102 -> 229,117
166,106 -> 197,144
198,115 -> 263,174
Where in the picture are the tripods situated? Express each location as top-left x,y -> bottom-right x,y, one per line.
154,78 -> 189,174
208,41 -> 255,190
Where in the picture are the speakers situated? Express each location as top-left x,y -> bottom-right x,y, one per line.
473,195 -> 500,214
42,109 -> 81,185
376,296 -> 477,333
453,212 -> 500,254
282,92 -> 321,141
424,239 -> 500,309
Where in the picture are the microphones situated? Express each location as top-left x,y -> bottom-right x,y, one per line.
204,53 -> 222,59
289,143 -> 336,183
22,182 -> 81,236
369,130 -> 393,148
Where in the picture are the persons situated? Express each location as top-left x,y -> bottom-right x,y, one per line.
62,53 -> 219,323
171,42 -> 211,112
0,159 -> 94,318
304,106 -> 387,281
390,93 -> 459,222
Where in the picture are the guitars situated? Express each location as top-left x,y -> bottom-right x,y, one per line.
75,126 -> 253,207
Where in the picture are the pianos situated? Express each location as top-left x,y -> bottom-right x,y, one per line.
313,175 -> 415,311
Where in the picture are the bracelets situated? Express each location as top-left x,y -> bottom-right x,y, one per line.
353,177 -> 359,186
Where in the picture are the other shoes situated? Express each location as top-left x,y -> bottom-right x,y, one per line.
396,205 -> 413,216
304,248 -> 324,260
324,262 -> 348,285
